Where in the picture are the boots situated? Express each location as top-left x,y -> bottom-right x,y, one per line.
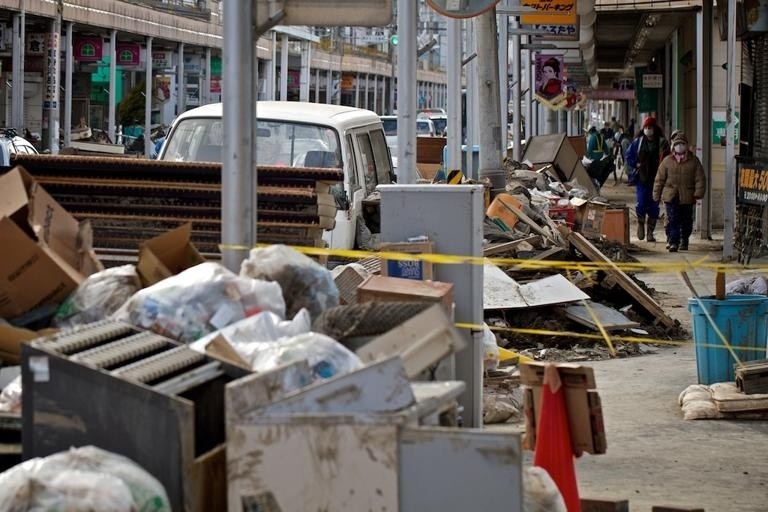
637,216 -> 656,241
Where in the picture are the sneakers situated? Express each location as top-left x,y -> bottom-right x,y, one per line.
679,244 -> 688,250
666,241 -> 678,252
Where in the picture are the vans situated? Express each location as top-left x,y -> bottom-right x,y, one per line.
155,101 -> 395,250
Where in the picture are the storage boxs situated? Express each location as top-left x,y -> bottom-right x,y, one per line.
358,276 -> 453,324
136,220 -> 206,287
1,169 -> 83,321
416,136 -> 446,181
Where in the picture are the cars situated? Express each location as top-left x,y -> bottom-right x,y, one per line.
376,107 -> 449,176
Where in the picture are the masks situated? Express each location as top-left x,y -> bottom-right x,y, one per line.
673,144 -> 686,153
643,129 -> 654,136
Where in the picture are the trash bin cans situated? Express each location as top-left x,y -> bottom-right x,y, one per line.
688,294 -> 768,386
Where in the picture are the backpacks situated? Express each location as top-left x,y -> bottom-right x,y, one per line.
620,136 -> 644,183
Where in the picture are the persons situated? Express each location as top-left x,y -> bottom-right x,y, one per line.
651,129 -> 707,255
580,115 -> 636,191
656,128 -> 687,249
621,115 -> 672,244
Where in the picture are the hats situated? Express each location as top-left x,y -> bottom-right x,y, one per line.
670,130 -> 689,152
643,116 -> 656,128
